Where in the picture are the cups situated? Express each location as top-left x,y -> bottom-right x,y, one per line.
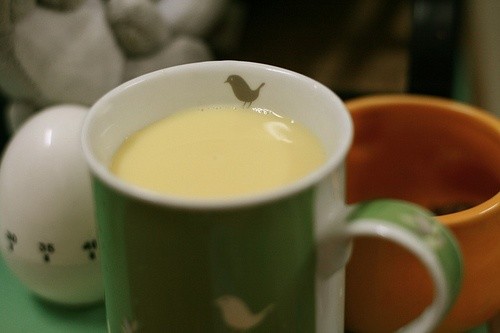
342,96 -> 500,332
79,58 -> 463,330
